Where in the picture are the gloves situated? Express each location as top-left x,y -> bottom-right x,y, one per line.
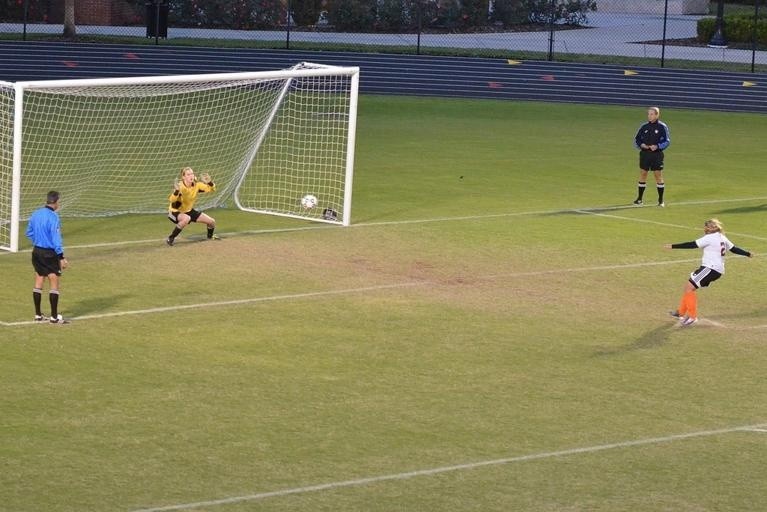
200,173 -> 211,184
174,178 -> 180,189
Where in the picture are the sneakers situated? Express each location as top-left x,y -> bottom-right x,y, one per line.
35,313 -> 50,321
682,317 -> 698,326
672,314 -> 683,320
167,236 -> 174,246
658,202 -> 664,208
207,235 -> 220,240
50,316 -> 71,324
634,200 -> 643,205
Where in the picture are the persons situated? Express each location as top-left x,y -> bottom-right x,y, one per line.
24,190 -> 72,326
633,106 -> 671,206
662,218 -> 754,328
164,167 -> 221,246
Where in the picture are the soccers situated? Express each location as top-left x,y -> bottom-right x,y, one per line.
301,195 -> 317,210
